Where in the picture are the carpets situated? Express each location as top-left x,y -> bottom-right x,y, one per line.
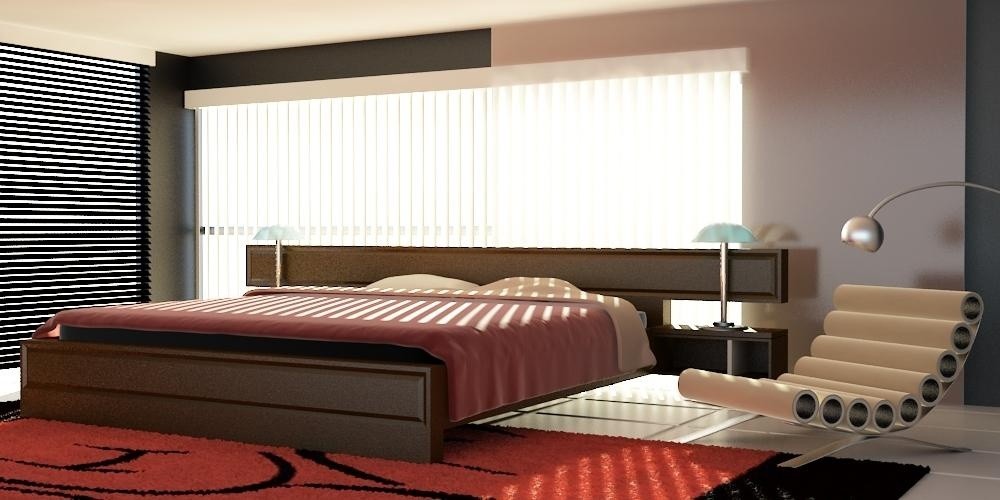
1,390 -> 932,499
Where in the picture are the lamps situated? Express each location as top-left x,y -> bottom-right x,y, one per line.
841,182 -> 1000,252
251,226 -> 303,287
693,223 -> 757,327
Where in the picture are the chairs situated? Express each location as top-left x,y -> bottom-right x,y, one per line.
675,284 -> 983,469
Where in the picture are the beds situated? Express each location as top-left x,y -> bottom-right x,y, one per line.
20,241 -> 792,464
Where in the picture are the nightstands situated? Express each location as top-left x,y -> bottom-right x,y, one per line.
645,323 -> 788,380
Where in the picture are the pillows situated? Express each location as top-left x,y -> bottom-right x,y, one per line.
365,273 -> 480,295
468,276 -> 582,299
